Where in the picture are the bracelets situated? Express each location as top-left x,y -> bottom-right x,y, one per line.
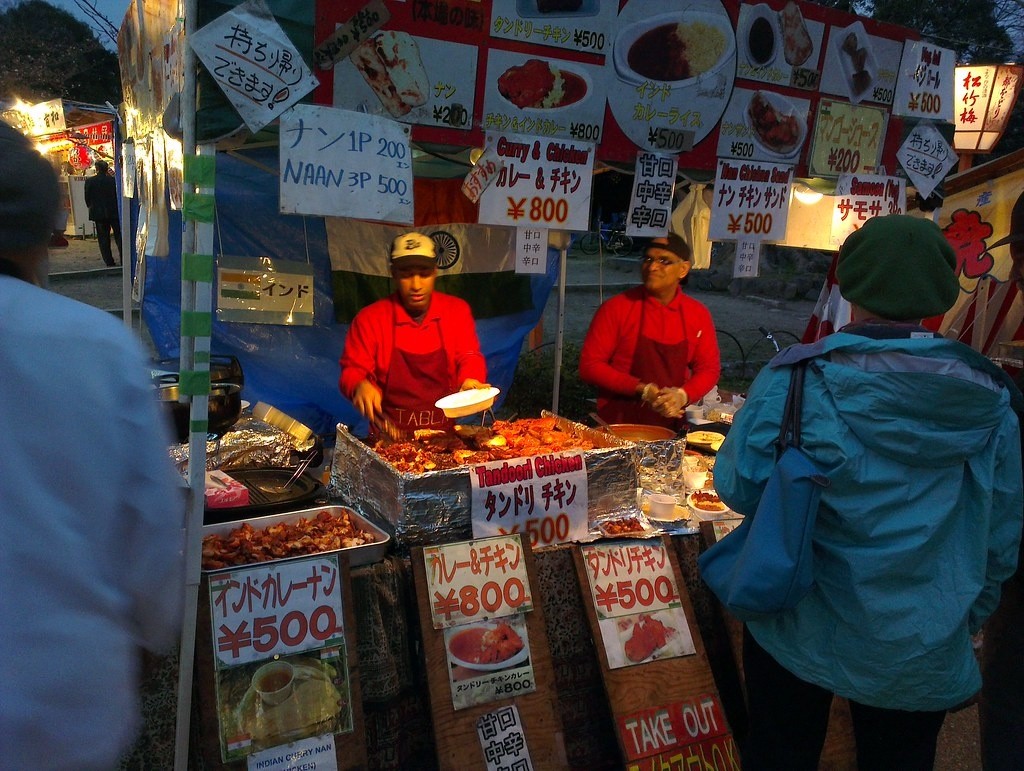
641,383 -> 659,402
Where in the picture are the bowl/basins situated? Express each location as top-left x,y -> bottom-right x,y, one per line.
685,405 -> 704,419
687,490 -> 730,521
684,456 -> 698,466
252,661 -> 295,706
435,387 -> 500,418
445,622 -> 528,671
682,466 -> 708,490
646,494 -> 677,520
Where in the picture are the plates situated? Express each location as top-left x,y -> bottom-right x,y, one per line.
687,431 -> 724,444
238,666 -> 330,734
595,517 -> 655,538
239,400 -> 251,409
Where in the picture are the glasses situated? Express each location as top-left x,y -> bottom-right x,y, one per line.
640,253 -> 685,268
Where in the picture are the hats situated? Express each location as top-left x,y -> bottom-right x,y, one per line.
644,232 -> 691,261
985,193 -> 1024,250
390,231 -> 435,269
836,215 -> 960,320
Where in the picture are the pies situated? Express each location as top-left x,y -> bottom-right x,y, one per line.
350,30 -> 431,119
782,2 -> 812,66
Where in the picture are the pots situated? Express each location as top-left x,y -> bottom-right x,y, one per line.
577,416 -> 686,442
153,374 -> 242,438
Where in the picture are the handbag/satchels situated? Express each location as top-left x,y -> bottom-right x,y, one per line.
698,358 -> 830,610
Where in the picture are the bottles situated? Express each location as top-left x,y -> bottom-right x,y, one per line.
324,465 -> 332,486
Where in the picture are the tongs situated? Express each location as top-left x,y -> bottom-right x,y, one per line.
374,412 -> 405,443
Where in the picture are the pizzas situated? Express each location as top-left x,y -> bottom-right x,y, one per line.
749,91 -> 801,154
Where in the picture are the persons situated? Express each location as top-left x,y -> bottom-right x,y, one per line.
712,215 -> 1023,771
84,160 -> 122,267
1,120 -> 185,770
980,193 -> 1022,771
580,233 -> 720,428
339,233 -> 487,437
